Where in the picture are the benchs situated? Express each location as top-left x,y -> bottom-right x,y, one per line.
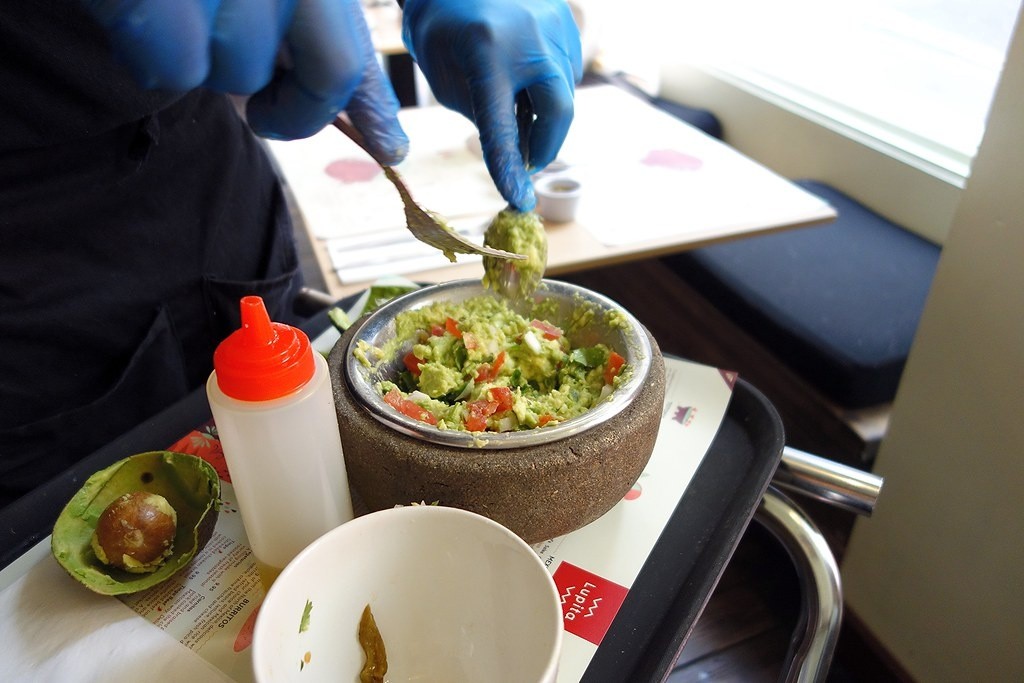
586,71 -> 943,453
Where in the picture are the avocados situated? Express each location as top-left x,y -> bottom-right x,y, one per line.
352,209 -> 626,428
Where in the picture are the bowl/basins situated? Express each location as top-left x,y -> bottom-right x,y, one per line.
250,505 -> 564,683
348,273 -> 652,448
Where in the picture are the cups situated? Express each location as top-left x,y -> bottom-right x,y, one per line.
535,176 -> 583,224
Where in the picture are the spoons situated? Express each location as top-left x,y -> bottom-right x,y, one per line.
484,92 -> 546,301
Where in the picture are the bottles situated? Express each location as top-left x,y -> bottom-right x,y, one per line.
207,297 -> 353,596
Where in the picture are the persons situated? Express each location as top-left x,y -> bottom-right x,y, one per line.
0,0 -> 584,510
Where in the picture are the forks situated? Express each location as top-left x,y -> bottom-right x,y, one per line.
331,116 -> 528,259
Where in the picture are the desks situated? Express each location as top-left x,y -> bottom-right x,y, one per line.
256,74 -> 844,283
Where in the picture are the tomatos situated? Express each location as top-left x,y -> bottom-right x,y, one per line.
529,319 -> 563,340
384,318 -> 513,431
605,352 -> 624,384
